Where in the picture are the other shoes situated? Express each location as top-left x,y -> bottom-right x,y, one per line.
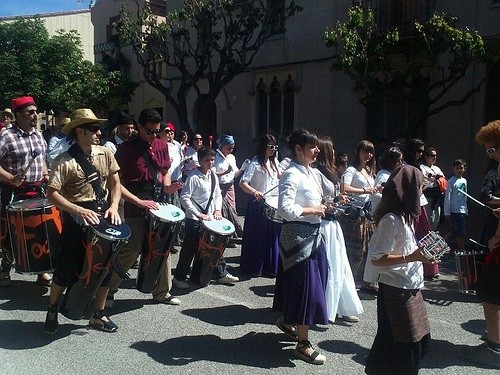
464,340 -> 500,369
341,315 -> 359,322
309,322 -> 329,331
0,246 -> 241,335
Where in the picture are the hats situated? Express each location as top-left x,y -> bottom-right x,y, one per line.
10,96 -> 37,113
166,122 -> 176,132
0,108 -> 14,120
61,108 -> 108,136
56,118 -> 72,128
219,135 -> 235,146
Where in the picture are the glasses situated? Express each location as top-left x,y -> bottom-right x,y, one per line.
164,129 -> 172,134
26,110 -> 39,115
425,154 -> 437,157
484,144 -> 500,155
193,138 -> 203,141
143,127 -> 161,135
81,125 -> 103,132
417,148 -> 422,153
267,145 -> 278,150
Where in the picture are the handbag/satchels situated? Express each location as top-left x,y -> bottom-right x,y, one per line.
254,198 -> 276,221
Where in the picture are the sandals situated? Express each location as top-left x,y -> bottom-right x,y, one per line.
293,339 -> 327,365
274,315 -> 298,341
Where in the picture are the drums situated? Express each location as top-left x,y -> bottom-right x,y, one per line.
58,211 -> 132,321
135,202 -> 185,294
454,248 -> 491,296
262,193 -> 284,224
5,197 -> 65,276
189,215 -> 236,287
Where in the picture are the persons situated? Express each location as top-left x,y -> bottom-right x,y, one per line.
0,96 -> 251,284
272,128 -> 334,364
171,147 -> 239,288
282,119 -> 500,347
363,168 -> 448,375
44,109 -> 122,334
105,108 -> 182,308
239,133 -> 286,277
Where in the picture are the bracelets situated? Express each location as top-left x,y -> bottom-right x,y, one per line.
402,255 -> 407,263
253,191 -> 256,195
363,188 -> 365,194
